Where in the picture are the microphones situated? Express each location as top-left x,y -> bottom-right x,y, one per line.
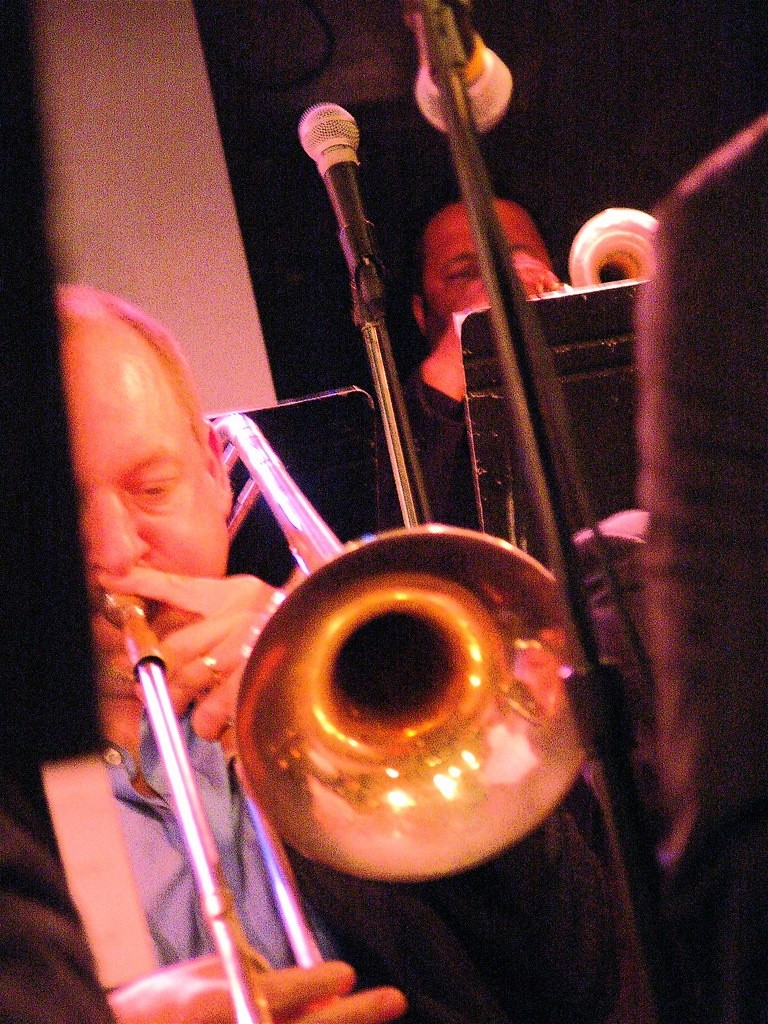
295,102 -> 388,321
401,1 -> 515,132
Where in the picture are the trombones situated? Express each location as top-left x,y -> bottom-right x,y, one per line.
98,412 -> 587,1024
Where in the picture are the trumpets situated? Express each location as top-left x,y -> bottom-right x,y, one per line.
570,206 -> 662,290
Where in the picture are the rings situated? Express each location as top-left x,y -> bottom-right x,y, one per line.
199,655 -> 227,684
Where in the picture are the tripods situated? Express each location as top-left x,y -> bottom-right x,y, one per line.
212,1 -> 698,1023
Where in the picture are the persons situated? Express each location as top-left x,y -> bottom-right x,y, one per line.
38,284 -> 408,1024
377,186 -> 563,528
